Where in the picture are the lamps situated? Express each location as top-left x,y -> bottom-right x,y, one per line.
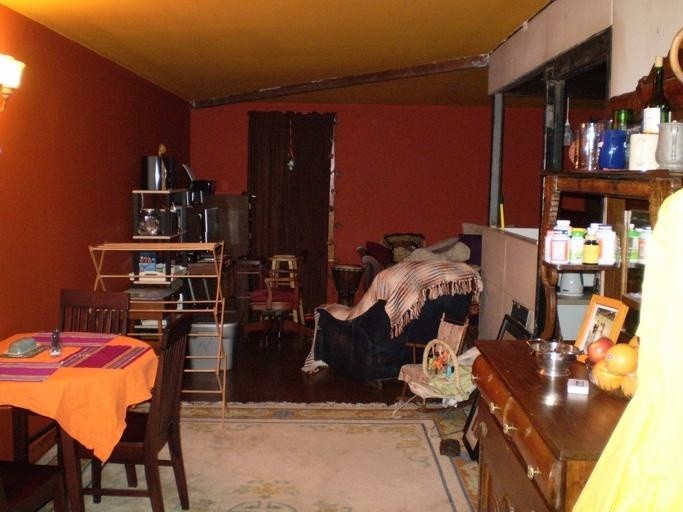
287,111 -> 297,171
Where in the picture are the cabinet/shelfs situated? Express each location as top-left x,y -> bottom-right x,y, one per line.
472,0 -> 683,512
130,188 -> 188,288
87,240 -> 232,434
189,192 -> 250,259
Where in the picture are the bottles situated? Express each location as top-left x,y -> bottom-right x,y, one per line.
645,55 -> 672,122
543,219 -> 653,267
49,329 -> 62,356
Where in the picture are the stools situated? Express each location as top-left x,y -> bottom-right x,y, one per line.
233,254 -> 263,316
269,253 -> 305,325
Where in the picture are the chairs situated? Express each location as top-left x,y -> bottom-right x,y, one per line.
249,255 -> 305,322
314,257 -> 473,383
395,313 -> 476,412
0,460 -> 67,512
55,313 -> 193,512
56,289 -> 131,337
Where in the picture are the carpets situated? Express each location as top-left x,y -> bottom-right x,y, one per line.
31,399 -> 483,512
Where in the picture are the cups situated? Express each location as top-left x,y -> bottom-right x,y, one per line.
536,342 -> 580,377
576,106 -> 683,171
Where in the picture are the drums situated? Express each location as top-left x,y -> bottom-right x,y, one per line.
331,262 -> 365,327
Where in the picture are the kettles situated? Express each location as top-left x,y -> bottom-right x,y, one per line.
559,273 -> 584,294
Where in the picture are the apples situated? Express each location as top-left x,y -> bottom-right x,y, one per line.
587,336 -> 615,363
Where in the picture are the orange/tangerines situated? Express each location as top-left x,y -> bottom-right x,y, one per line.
603,343 -> 637,377
592,359 -> 622,392
620,373 -> 636,398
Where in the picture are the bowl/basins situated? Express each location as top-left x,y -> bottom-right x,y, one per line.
584,357 -> 636,401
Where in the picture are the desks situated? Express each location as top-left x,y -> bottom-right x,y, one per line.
122,288 -> 180,344
0,330 -> 157,512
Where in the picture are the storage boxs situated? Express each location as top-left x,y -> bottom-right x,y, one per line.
189,322 -> 240,372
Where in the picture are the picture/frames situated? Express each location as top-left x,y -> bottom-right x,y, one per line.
575,293 -> 630,364
460,312 -> 533,459
